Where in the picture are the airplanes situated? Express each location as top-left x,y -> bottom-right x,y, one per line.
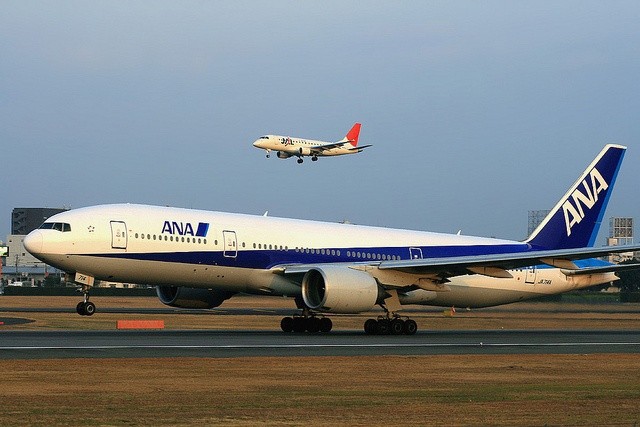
396,258 -> 640,310
254,122 -> 373,164
21,143 -> 640,337
